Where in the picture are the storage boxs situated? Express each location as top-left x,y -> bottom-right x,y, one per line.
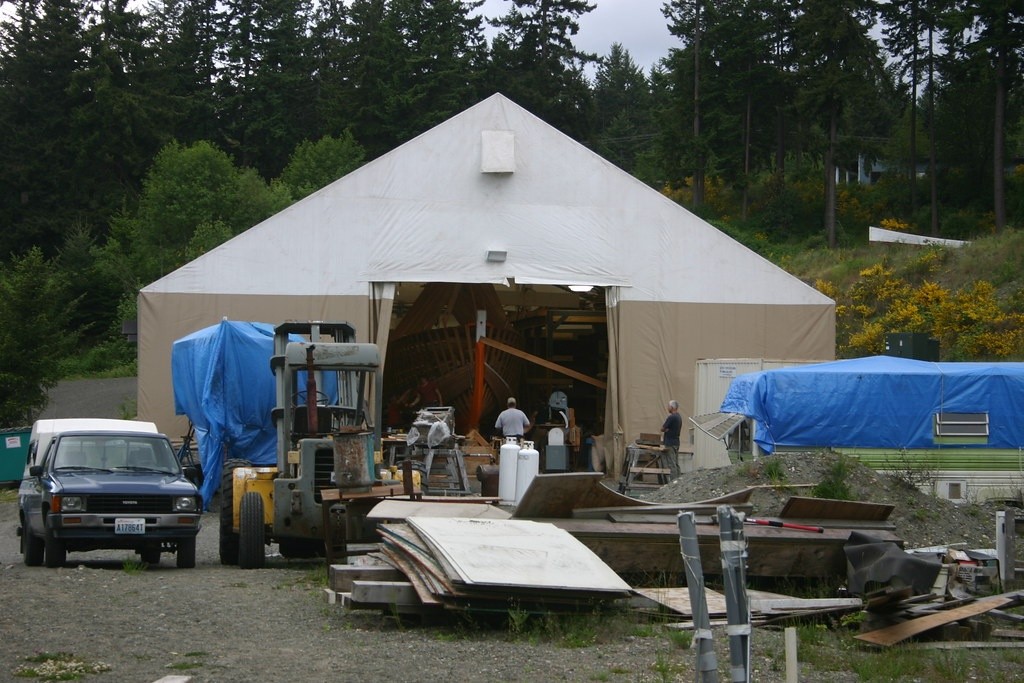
958,565 -> 999,596
640,433 -> 661,446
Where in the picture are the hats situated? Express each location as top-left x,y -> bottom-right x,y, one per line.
508,397 -> 516,404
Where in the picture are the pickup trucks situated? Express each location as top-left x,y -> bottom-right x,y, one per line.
14,417 -> 204,568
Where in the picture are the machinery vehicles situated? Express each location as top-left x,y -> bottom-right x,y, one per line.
219,318 -> 429,569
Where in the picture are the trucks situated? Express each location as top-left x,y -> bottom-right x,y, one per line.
686,354 -> 1024,503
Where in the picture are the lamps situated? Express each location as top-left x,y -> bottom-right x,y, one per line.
486,246 -> 508,263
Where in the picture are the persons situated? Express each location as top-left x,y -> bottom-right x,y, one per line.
396,375 -> 443,410
661,400 -> 682,481
495,397 -> 531,442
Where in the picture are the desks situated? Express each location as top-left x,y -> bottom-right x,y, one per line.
624,448 -> 670,488
319,484 -> 423,586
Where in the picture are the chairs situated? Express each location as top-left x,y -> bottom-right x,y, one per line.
64,451 -> 86,467
130,448 -> 156,467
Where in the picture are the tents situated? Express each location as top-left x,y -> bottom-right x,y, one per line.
134,90 -> 837,482
